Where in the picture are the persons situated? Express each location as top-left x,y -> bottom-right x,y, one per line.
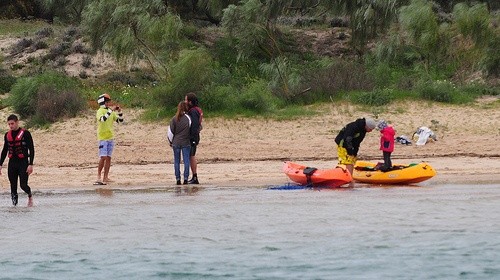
96,94 -> 124,185
0,114 -> 35,207
378,120 -> 395,172
167,92 -> 203,185
335,118 -> 376,177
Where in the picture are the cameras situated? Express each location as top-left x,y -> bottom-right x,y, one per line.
115,107 -> 119,110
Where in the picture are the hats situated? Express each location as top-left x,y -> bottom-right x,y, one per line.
103,93 -> 110,99
366,118 -> 376,129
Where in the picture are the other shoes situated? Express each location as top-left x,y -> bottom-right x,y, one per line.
93,182 -> 105,185
188,178 -> 193,182
183,180 -> 188,184
188,176 -> 199,184
177,180 -> 181,184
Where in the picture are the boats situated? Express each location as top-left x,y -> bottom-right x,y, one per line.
283,160 -> 353,188
337,163 -> 436,184
